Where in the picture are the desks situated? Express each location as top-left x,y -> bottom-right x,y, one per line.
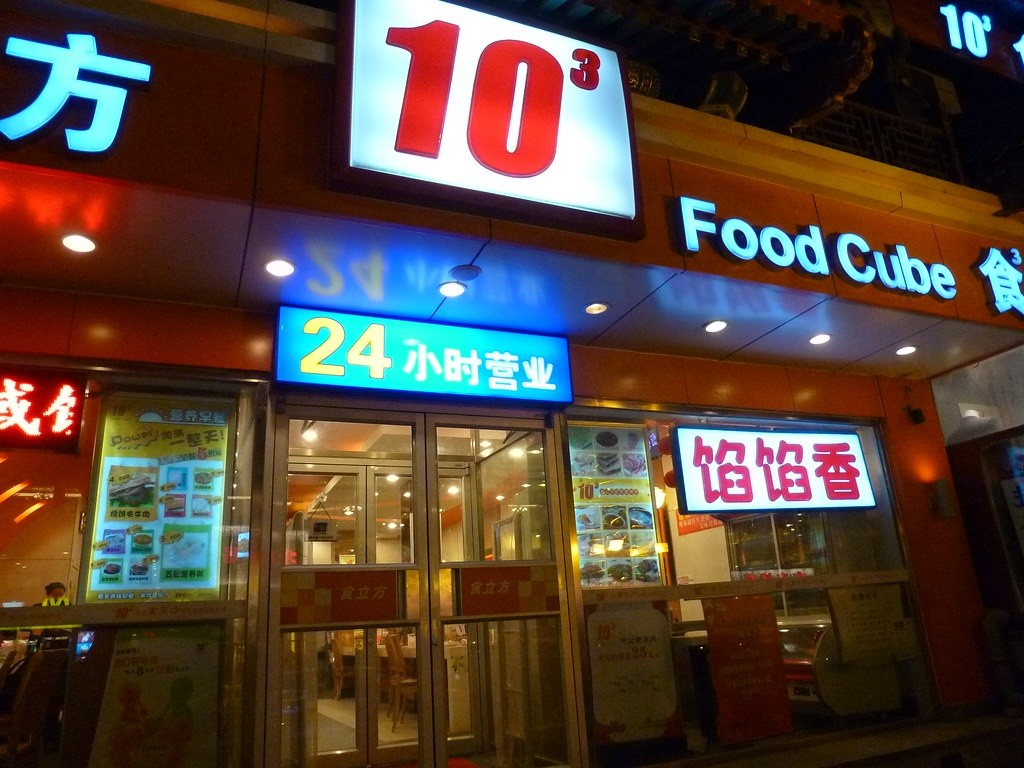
342,645 -> 471,736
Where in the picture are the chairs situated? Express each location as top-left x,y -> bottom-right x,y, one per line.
381,636 -> 417,732
329,639 -> 355,700
0,628 -> 72,768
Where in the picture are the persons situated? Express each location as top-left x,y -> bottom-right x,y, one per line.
20,582 -> 72,641
